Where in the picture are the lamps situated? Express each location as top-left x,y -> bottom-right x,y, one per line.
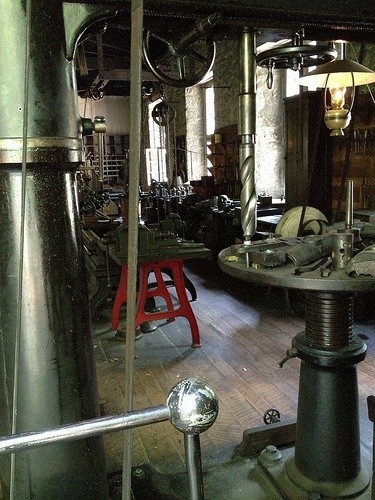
296,60 -> 375,136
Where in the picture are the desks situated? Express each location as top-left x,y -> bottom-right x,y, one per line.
219,236 -> 375,497
98,236 -> 212,348
